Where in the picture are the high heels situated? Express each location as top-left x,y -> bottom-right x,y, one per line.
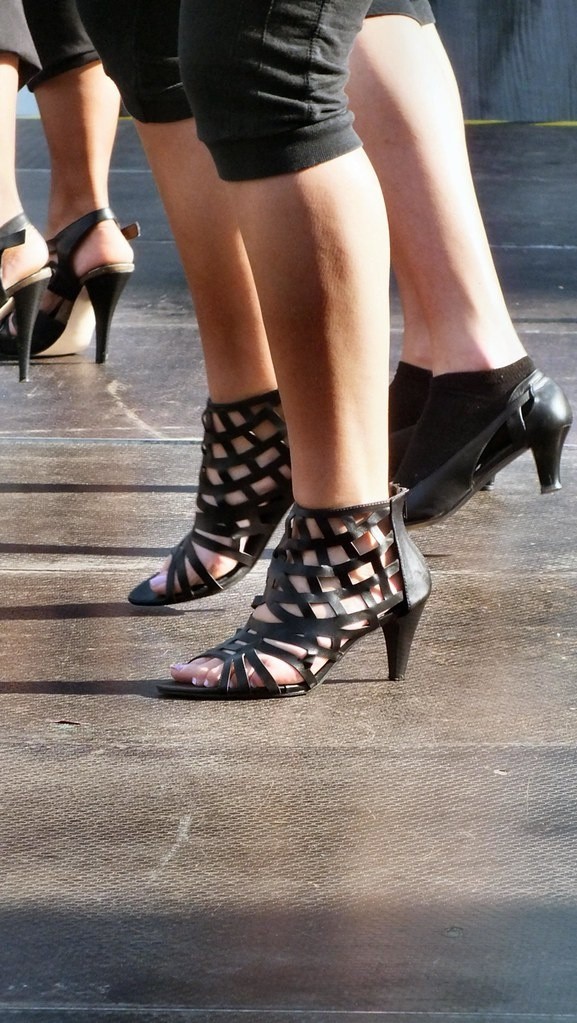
1,208 -> 141,382
127,388 -> 295,608
153,480 -> 433,698
389,366 -> 572,530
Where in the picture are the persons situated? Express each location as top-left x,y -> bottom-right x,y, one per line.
313,1 -> 577,545
0,1 -> 145,387
77,1 -> 430,706
